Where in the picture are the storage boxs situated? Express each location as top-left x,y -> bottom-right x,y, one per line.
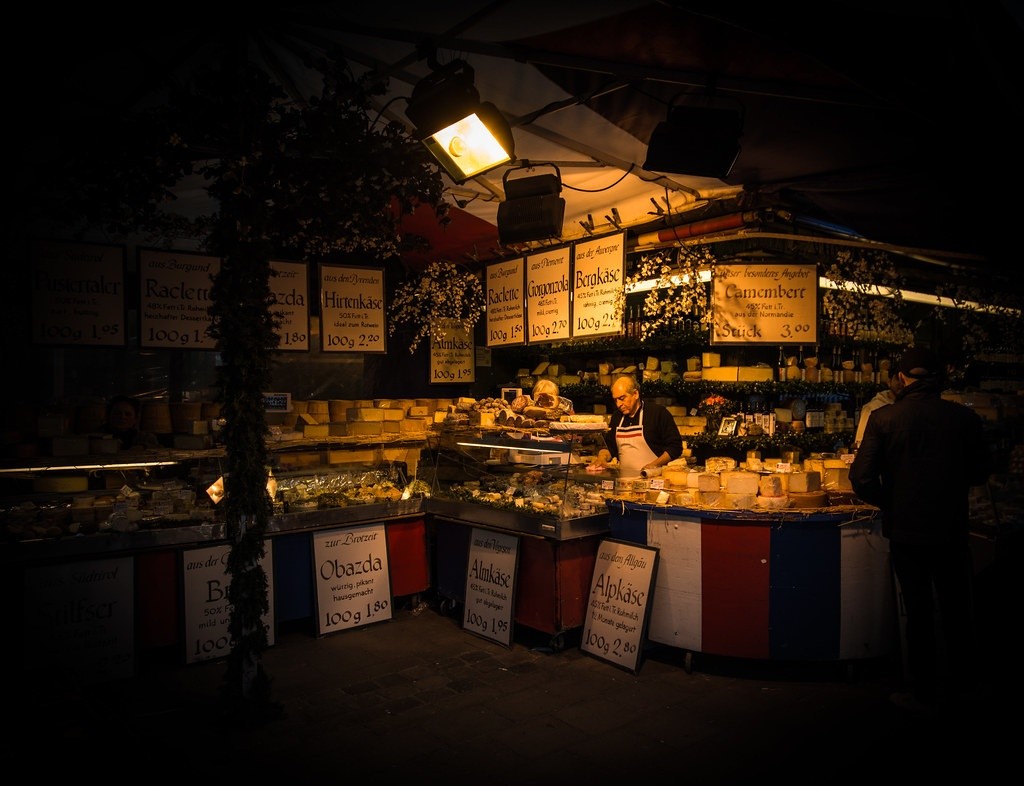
410,406 -> 428,416
470,411 -> 495,426
703,367 -> 774,383
399,419 -> 426,431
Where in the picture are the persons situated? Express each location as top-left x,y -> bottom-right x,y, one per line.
532,379 -> 560,401
848,345 -> 992,707
598,376 -> 682,479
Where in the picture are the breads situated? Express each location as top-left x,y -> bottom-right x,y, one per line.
260,398 -> 493,434
517,351 -> 896,511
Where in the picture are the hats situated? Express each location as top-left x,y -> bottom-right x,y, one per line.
899,345 -> 937,379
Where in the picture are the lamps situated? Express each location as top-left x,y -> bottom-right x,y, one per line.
497,162 -> 566,248
404,59 -> 517,185
643,107 -> 744,179
